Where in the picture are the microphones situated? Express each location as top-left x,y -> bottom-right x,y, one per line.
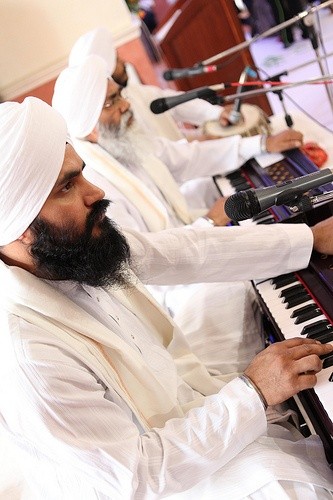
230,71 -> 248,123
163,66 -> 216,80
150,85 -> 224,115
223,169 -> 333,221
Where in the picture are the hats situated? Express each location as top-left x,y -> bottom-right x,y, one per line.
52,62 -> 109,138
68,29 -> 117,77
0,96 -> 68,246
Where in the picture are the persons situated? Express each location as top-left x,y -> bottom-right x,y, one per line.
50,55 -> 303,386
67,30 -> 244,233
0,95 -> 333,500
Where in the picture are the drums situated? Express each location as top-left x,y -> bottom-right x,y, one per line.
205,102 -> 271,139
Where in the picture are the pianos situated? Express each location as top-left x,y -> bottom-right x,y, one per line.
204,134 -> 332,475
